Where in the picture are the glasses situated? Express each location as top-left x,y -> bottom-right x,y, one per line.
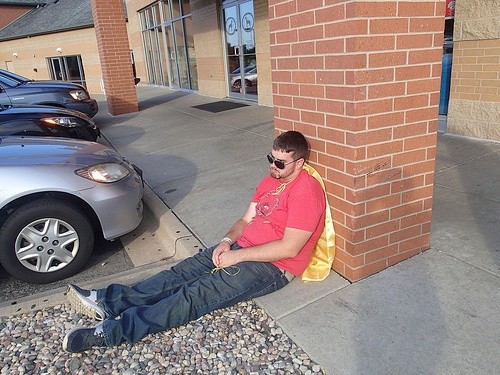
267,152 -> 303,170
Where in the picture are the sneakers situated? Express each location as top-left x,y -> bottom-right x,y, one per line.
67,283 -> 106,321
62,317 -> 110,353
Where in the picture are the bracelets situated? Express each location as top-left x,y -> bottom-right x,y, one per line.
221,237 -> 233,246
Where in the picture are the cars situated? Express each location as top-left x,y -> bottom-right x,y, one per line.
0,68 -> 87,90
0,135 -> 145,285
0,103 -> 102,142
0,76 -> 99,119
228,65 -> 258,89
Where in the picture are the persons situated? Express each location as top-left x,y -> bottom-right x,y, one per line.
62,131 -> 326,353
